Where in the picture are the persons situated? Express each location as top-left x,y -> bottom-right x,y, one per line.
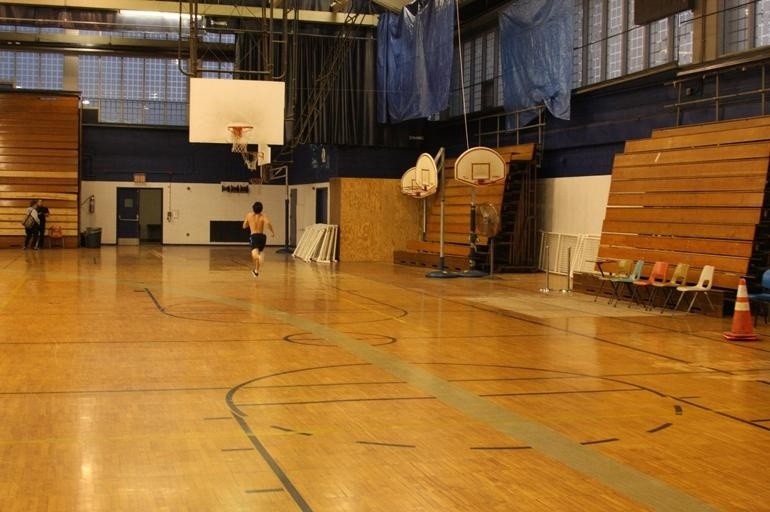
23,199 -> 50,250
242,202 -> 274,277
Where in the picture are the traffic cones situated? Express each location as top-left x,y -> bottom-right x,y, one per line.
722,277 -> 761,341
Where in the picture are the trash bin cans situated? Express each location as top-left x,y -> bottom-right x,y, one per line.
86,227 -> 101,248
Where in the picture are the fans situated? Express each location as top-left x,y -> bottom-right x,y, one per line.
475,203 -> 503,279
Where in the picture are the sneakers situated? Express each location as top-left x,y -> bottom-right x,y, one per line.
252,269 -> 258,277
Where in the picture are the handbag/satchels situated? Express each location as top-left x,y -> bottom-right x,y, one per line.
22,215 -> 36,230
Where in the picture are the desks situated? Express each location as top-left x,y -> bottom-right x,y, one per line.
585,259 -> 620,302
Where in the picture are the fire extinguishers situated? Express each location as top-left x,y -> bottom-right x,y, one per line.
81,195 -> 95,213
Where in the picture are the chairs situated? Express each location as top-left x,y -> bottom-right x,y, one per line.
48,225 -> 64,249
741,270 -> 770,327
599,259 -> 716,319
